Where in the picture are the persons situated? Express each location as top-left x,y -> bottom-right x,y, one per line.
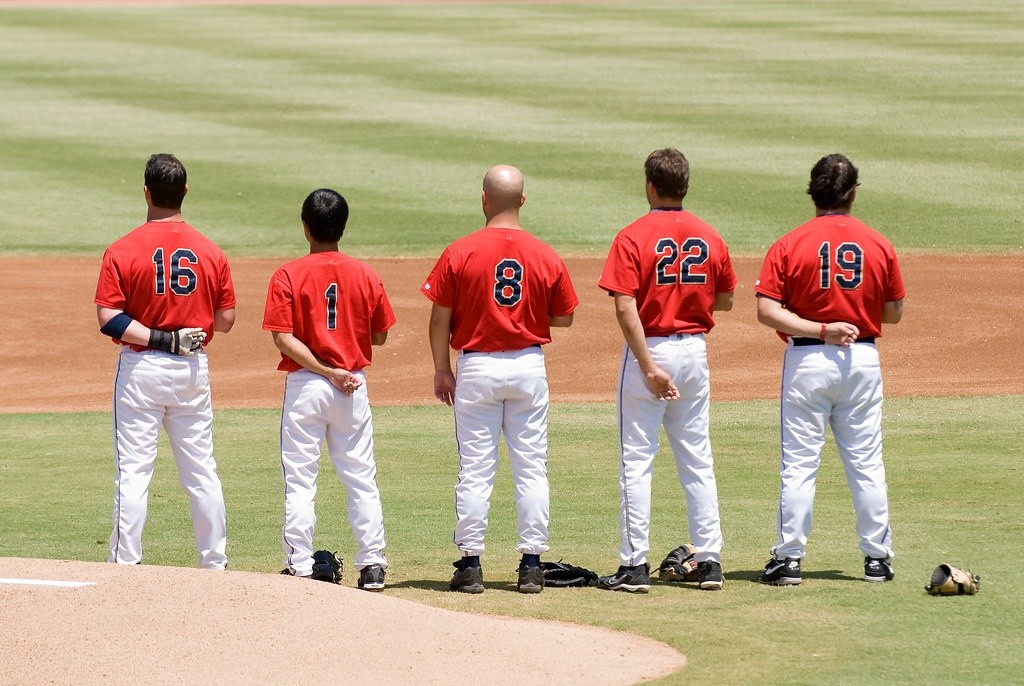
599,147 -> 738,593
420,164 -> 580,595
94,152 -> 237,567
755,152 -> 904,586
263,189 -> 399,593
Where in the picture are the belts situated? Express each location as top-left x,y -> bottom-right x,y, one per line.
663,332 -> 702,337
464,343 -> 541,354
792,335 -> 875,346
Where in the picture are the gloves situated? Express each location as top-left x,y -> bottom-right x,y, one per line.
168,327 -> 207,356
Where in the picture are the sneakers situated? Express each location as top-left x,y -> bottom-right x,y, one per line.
358,565 -> 386,591
515,560 -> 545,593
863,555 -> 895,583
758,557 -> 802,585
697,560 -> 724,589
597,563 -> 650,594
278,567 -> 312,579
449,564 -> 484,593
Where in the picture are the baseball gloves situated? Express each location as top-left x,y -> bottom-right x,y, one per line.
658,544 -> 702,583
540,559 -> 597,588
927,563 -> 979,597
311,549 -> 345,584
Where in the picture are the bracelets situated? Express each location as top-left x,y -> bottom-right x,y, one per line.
820,323 -> 826,341
